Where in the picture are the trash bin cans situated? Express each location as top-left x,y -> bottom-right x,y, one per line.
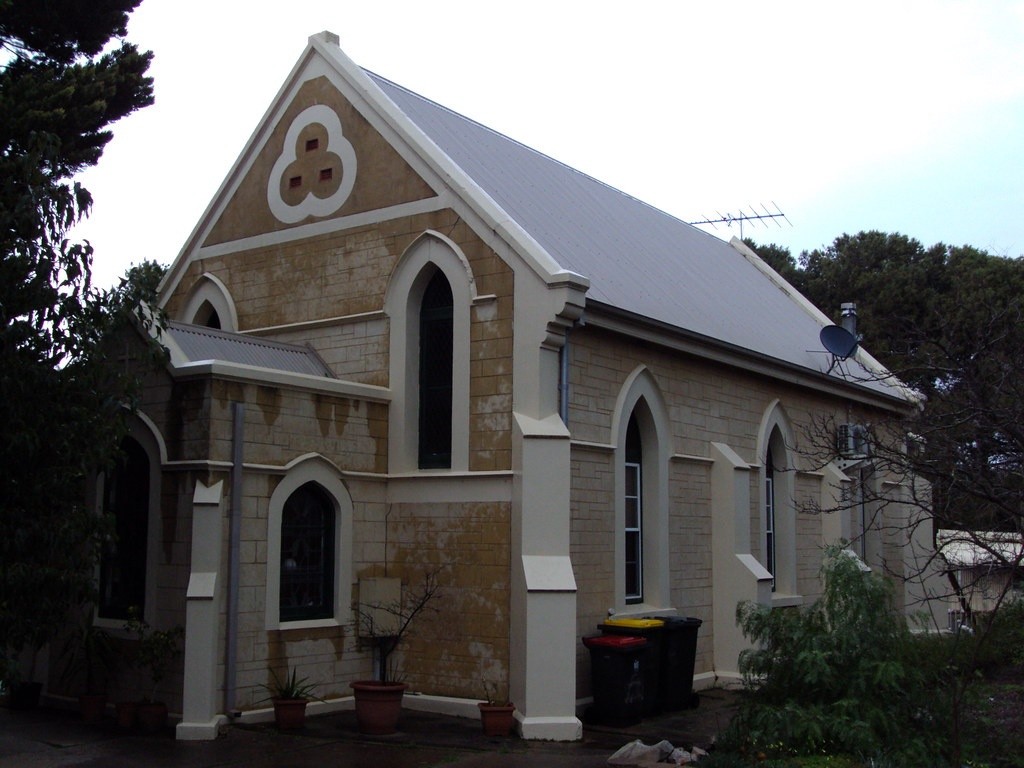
581,632 -> 650,727
648,615 -> 704,709
597,617 -> 666,712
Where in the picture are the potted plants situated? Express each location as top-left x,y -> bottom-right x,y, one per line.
52,600 -> 185,735
478,679 -> 516,737
344,567 -> 440,735
252,665 -> 329,730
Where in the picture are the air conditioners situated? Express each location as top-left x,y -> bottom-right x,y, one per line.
837,423 -> 870,457
